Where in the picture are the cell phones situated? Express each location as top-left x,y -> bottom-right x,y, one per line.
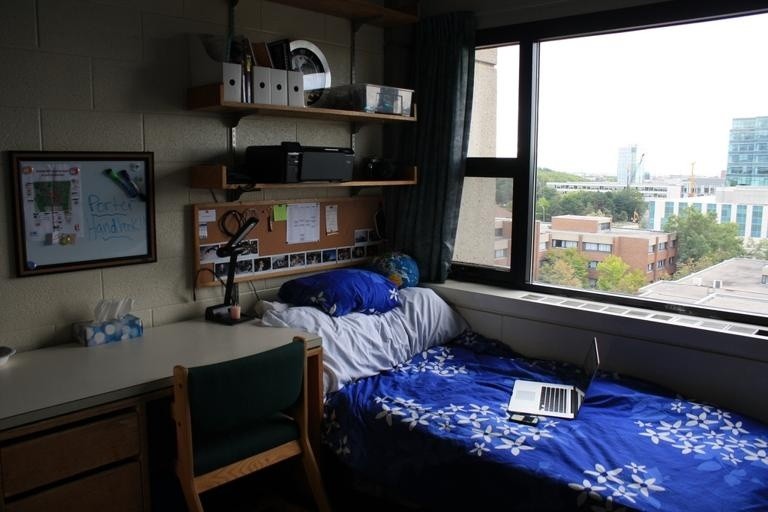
509,413 -> 539,426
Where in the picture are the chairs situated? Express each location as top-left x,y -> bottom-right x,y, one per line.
164,335 -> 332,511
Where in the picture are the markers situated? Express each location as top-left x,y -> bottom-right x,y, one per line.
107,168 -> 146,203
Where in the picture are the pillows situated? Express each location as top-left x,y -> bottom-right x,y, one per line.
256,267 -> 473,403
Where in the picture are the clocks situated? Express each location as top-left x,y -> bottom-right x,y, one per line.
289,40 -> 331,108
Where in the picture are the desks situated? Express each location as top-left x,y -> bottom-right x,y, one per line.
1,313 -> 323,512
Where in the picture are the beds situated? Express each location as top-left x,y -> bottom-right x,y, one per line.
323,332 -> 768,511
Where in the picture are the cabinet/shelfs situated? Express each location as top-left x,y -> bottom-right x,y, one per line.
187,0 -> 421,190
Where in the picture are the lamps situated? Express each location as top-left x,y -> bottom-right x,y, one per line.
204,216 -> 259,326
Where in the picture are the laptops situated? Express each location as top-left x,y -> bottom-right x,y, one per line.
507,335 -> 600,420
